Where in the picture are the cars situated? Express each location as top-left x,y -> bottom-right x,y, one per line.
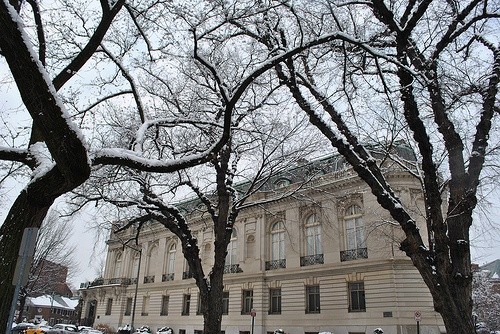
10,323 -> 102,334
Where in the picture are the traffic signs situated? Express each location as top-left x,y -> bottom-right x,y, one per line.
251,309 -> 256,316
415,311 -> 422,321
